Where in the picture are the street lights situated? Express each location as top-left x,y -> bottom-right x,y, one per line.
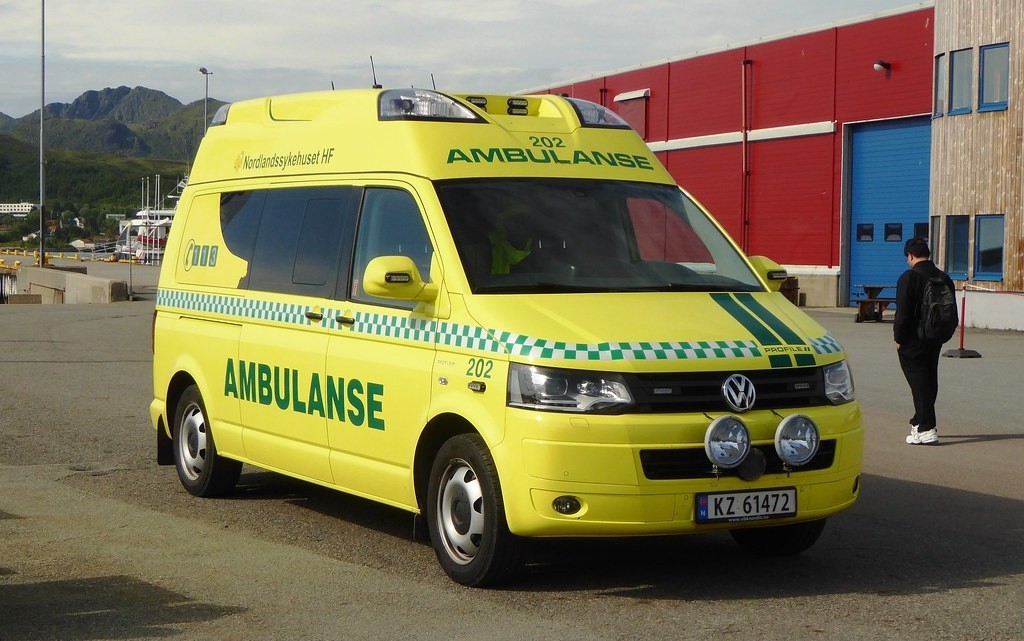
198,67 -> 208,135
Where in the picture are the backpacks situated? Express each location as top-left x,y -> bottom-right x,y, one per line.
910,269 -> 958,346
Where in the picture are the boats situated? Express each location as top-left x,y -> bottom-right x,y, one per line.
114,209 -> 171,260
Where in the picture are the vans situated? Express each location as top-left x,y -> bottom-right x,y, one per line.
146,85 -> 864,587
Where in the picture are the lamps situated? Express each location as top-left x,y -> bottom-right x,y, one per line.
873,60 -> 890,71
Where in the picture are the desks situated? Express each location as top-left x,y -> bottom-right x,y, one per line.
852,285 -> 897,299
784,277 -> 796,287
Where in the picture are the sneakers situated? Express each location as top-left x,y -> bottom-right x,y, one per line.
905,424 -> 939,445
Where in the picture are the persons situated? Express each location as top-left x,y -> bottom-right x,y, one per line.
488,203 -> 537,276
893,236 -> 955,445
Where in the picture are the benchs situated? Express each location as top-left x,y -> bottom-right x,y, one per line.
780,288 -> 799,291
848,299 -> 896,323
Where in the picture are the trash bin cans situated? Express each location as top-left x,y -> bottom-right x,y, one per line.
779,277 -> 799,306
